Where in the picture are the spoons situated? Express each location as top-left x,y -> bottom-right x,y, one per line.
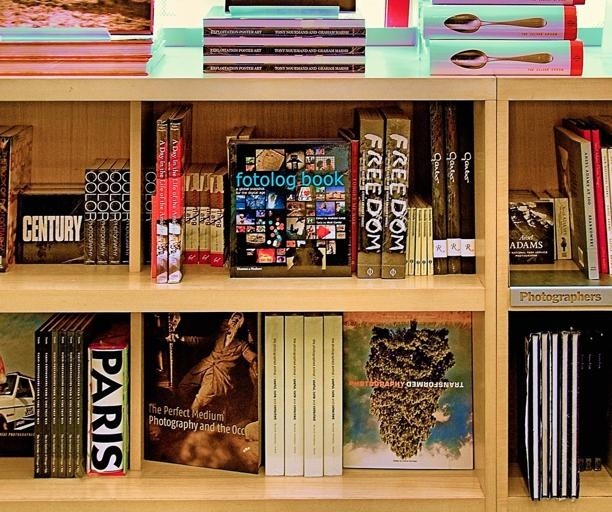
444,13 -> 547,33
450,50 -> 553,70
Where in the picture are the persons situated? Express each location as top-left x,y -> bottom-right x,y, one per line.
170,312 -> 258,420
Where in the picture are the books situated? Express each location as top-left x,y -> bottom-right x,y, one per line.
181,161 -> 228,266
225,125 -> 359,277
520,324 -> 582,501
413,0 -> 587,79
141,310 -> 261,477
352,104 -> 411,279
0,27 -> 155,77
406,196 -> 432,276
261,315 -> 345,479
144,101 -> 193,284
0,125 -> 130,273
413,103 -> 475,275
344,312 -> 474,470
199,0 -> 368,75
508,114 -> 612,280
29,313 -> 130,477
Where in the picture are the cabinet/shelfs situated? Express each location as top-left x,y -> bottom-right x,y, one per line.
496,28 -> 611,511
1,27 -> 496,512
